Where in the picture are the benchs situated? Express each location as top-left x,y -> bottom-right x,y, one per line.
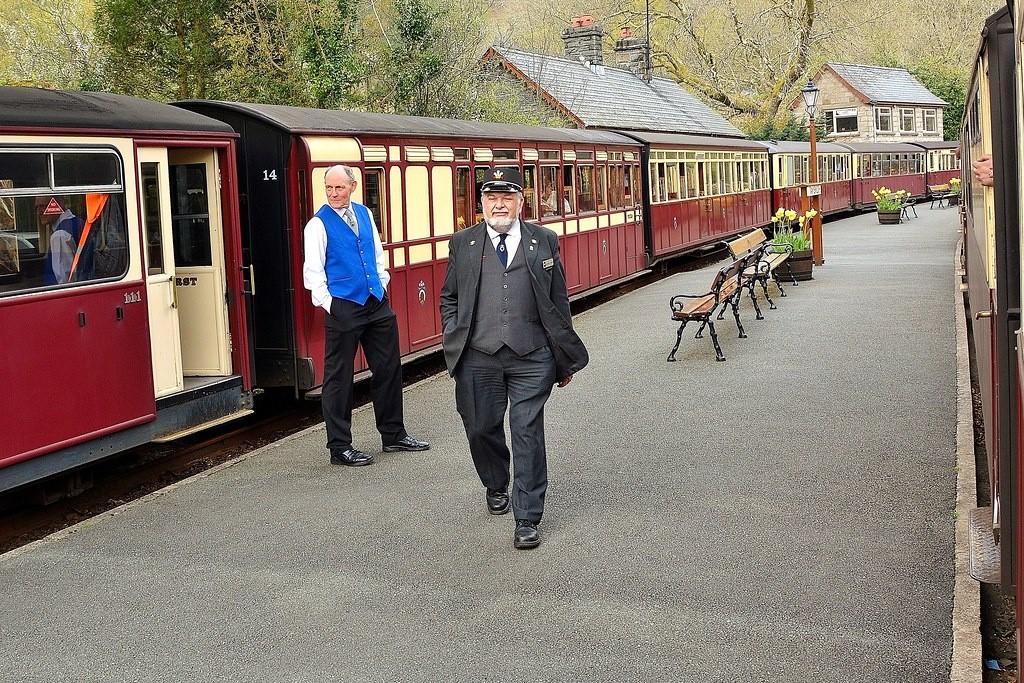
882,193 -> 918,223
667,246 -> 764,362
720,227 -> 798,310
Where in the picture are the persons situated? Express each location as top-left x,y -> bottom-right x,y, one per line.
439,168 -> 589,548
302,165 -> 431,466
540,178 -> 571,216
34,191 -> 95,285
972,154 -> 993,187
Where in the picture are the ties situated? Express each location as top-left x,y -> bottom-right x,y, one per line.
345,209 -> 358,236
496,234 -> 509,269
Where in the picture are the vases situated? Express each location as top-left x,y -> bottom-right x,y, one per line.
770,250 -> 814,282
878,208 -> 901,224
948,196 -> 961,206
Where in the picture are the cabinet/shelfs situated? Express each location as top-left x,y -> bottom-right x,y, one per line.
927,184 -> 958,210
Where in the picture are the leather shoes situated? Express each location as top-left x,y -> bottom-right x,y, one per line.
383,436 -> 430,452
331,447 -> 374,466
486,471 -> 511,514
514,519 -> 540,547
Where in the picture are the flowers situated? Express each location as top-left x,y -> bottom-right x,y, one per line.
949,177 -> 961,193
871,185 -> 911,210
772,206 -> 818,252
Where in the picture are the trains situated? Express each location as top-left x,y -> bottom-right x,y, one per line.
0,84 -> 959,494
960,2 -> 1024,676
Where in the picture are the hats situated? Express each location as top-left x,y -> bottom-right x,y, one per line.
482,168 -> 524,193
